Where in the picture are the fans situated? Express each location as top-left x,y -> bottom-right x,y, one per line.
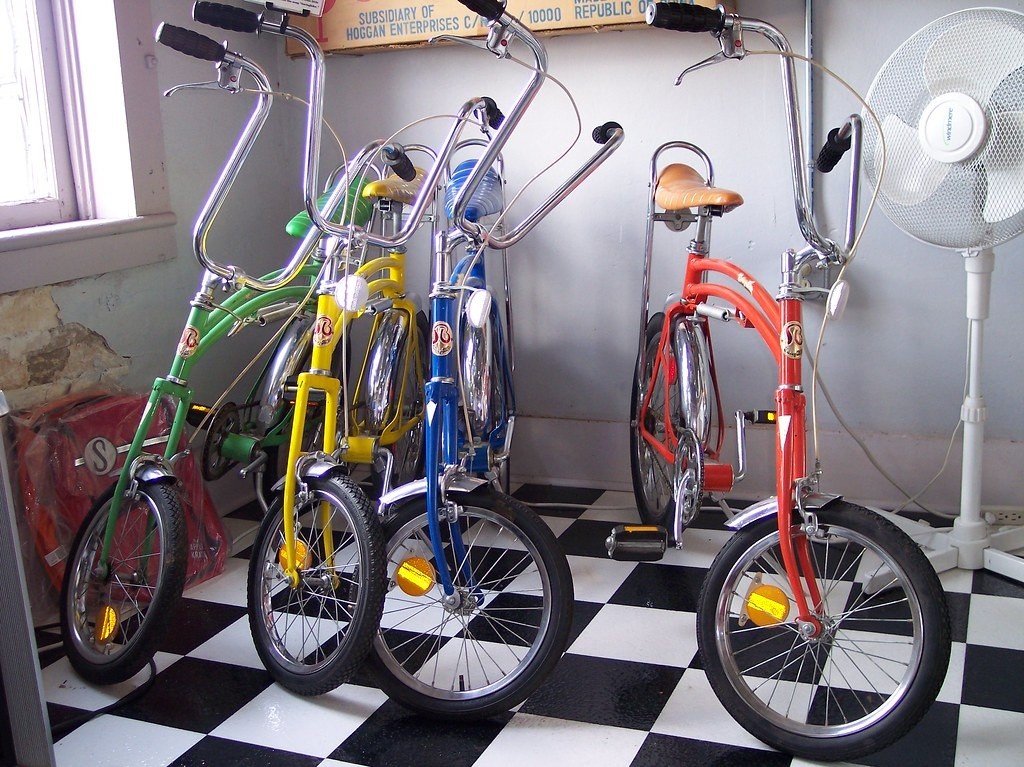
830,5 -> 1024,594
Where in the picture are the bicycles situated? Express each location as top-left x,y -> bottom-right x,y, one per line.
64,1 -> 577,724
625,0 -> 951,765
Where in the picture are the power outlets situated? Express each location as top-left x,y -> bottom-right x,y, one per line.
980,509 -> 1024,528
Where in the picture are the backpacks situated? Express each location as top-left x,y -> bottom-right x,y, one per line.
5,388 -> 229,605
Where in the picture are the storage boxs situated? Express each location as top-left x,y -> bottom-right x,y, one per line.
284,0 -> 738,60
